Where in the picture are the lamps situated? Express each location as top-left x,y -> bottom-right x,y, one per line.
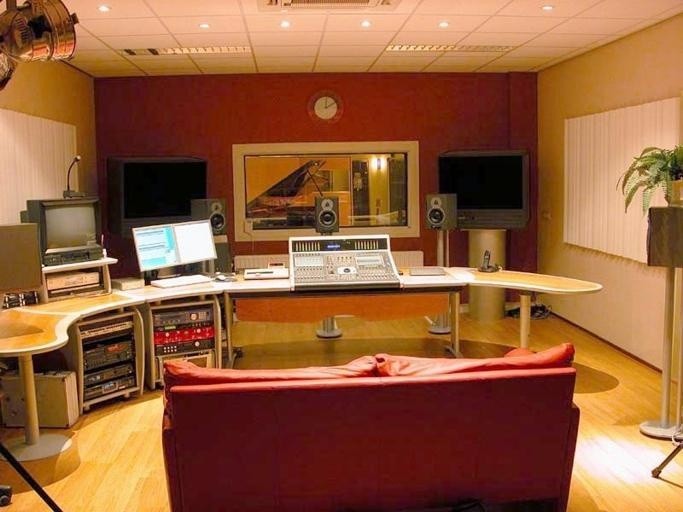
0,0 -> 79,92
638,207 -> 683,441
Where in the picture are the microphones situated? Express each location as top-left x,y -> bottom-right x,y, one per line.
63,154 -> 81,196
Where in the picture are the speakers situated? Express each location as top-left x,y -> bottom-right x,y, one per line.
315,195 -> 340,232
424,193 -> 458,231
190,197 -> 227,235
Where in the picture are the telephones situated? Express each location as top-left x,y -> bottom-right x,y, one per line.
478,250 -> 500,272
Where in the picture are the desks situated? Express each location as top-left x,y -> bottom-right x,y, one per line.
0,267 -> 603,464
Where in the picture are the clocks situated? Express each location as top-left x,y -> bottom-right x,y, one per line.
307,89 -> 343,127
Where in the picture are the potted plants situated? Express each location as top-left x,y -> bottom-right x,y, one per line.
616,145 -> 683,220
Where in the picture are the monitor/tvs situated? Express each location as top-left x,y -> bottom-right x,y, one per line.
20,196 -> 104,267
173,220 -> 218,269
131,223 -> 180,279
437,149 -> 530,229
107,154 -> 208,241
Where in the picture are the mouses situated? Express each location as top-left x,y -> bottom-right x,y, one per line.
216,275 -> 226,281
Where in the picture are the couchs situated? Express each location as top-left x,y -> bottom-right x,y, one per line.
162,342 -> 580,511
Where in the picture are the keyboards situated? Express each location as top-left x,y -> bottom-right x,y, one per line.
150,274 -> 210,287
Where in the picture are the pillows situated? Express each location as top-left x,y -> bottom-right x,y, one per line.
374,341 -> 576,376
162,356 -> 376,417
